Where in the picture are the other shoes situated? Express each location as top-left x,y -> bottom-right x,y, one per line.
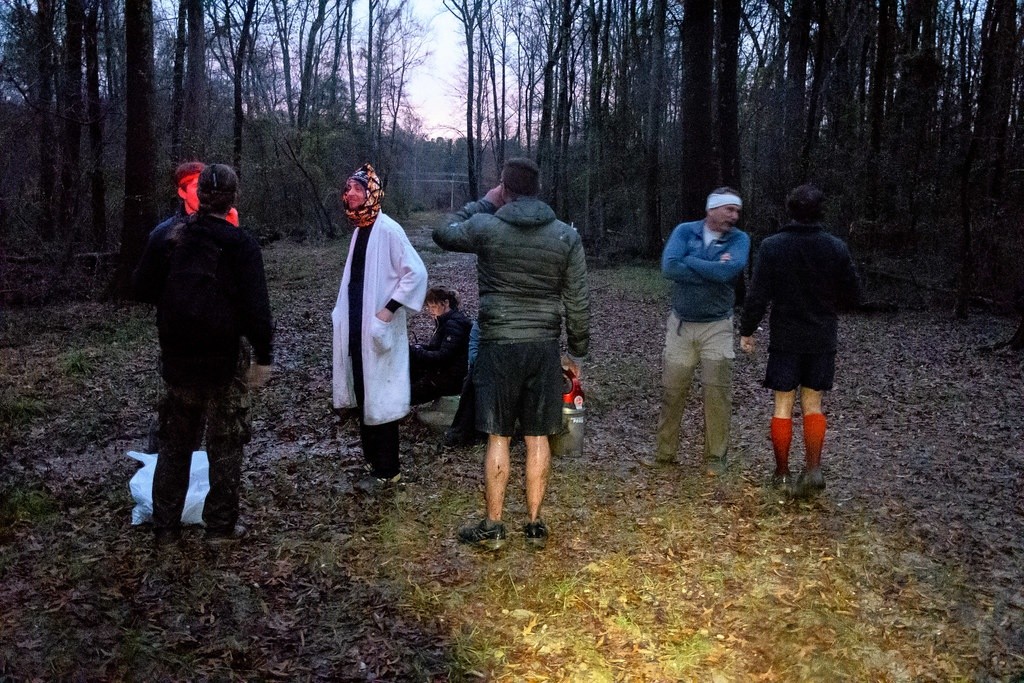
793,465 -> 825,487
765,474 -> 792,494
156,523 -> 181,549
645,453 -> 678,467
706,453 -> 726,475
204,524 -> 246,544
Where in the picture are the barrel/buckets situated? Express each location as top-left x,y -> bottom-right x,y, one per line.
546,366 -> 585,460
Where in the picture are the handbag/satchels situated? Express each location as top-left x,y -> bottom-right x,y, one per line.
127,450 -> 211,526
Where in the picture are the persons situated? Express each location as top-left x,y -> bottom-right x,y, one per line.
408,288 -> 471,404
128,160 -> 274,547
653,187 -> 750,476
433,158 -> 590,550
442,321 -> 490,449
738,186 -> 864,488
330,163 -> 429,490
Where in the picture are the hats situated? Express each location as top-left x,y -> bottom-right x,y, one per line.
197,163 -> 237,197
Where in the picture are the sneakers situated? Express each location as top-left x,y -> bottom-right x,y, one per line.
457,518 -> 506,550
522,514 -> 548,548
359,462 -> 376,475
376,472 -> 402,485
